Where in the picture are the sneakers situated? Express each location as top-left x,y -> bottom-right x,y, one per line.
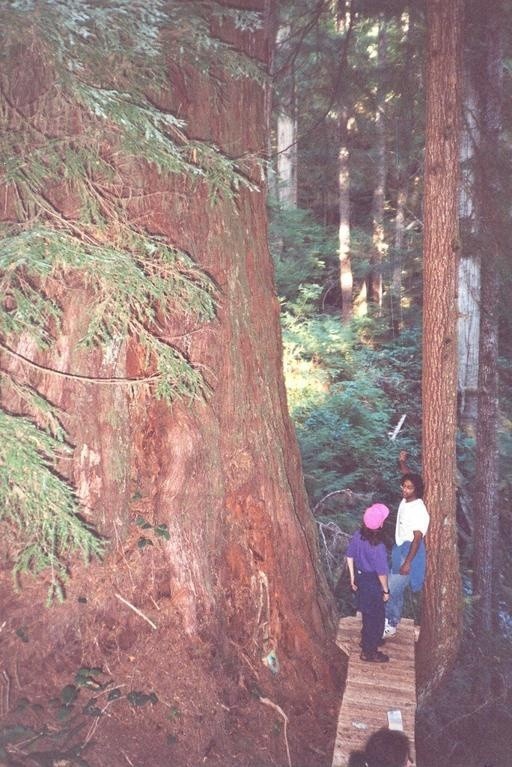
382,618 -> 398,640
360,651 -> 389,663
358,638 -> 385,648
413,625 -> 420,642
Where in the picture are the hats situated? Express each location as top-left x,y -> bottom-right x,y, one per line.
363,502 -> 390,530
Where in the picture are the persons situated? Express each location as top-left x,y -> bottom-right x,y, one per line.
381,451 -> 430,643
349,728 -> 413,767
346,503 -> 390,662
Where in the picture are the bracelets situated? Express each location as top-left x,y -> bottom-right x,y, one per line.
383,589 -> 390,594
